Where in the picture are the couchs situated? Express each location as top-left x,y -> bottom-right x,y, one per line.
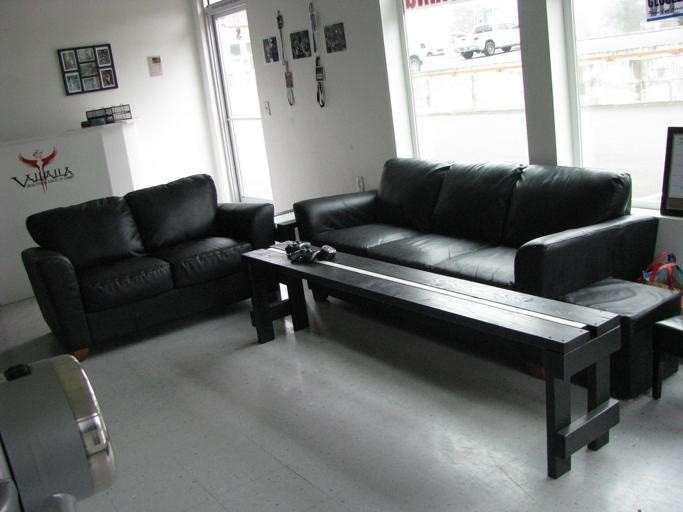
21,173 -> 274,363
292,158 -> 658,302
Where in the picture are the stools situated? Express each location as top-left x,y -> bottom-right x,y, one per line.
651,314 -> 683,400
561,275 -> 681,401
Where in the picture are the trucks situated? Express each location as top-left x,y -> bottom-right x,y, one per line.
409,41 -> 444,73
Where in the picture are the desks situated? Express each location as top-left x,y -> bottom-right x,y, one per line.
241,240 -> 621,478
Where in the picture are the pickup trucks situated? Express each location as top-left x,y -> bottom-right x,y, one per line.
458,21 -> 521,59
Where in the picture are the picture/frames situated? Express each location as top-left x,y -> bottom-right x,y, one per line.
57,44 -> 118,95
660,127 -> 683,216
